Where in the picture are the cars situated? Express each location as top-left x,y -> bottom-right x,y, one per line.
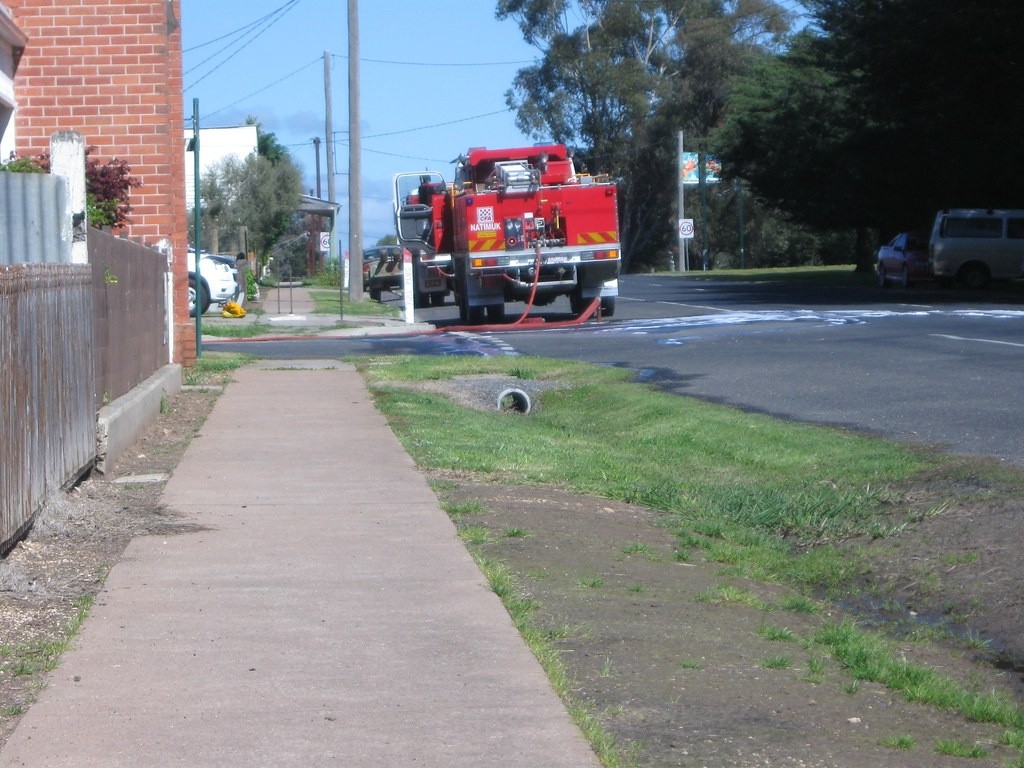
187,245 -> 239,318
876,229 -> 937,289
363,246 -> 402,292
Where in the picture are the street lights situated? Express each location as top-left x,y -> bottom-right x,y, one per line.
187,138 -> 202,356
313,137 -> 321,198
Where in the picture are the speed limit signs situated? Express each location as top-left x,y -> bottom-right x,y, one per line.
320,232 -> 331,252
679,219 -> 694,238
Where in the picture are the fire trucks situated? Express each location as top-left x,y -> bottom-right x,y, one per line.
392,146 -> 621,326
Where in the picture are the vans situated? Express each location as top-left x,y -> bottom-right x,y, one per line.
928,207 -> 1024,289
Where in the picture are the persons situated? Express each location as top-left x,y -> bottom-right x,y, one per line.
234,252 -> 248,308
